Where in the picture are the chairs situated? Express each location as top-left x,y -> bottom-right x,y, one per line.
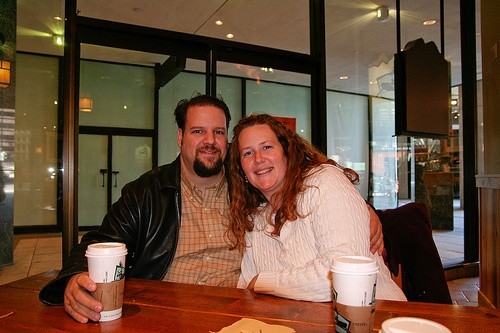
376,200 -> 451,302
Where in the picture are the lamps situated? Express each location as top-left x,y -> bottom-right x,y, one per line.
0,59 -> 11,89
80,91 -> 94,113
51,33 -> 65,47
376,6 -> 390,23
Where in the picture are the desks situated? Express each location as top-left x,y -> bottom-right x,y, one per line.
425,171 -> 454,231
0,268 -> 500,333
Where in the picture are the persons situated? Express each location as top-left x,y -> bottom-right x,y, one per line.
227,114 -> 409,302
39,93 -> 384,324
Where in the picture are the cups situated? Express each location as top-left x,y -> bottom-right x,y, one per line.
85,243 -> 128,321
330,257 -> 380,333
